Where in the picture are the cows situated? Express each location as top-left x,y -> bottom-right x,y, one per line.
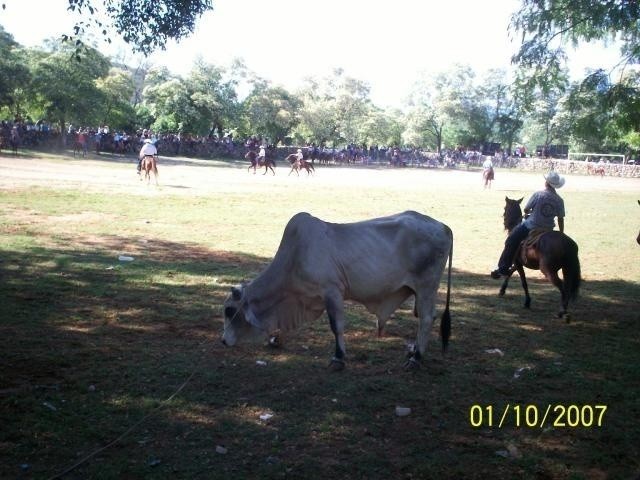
221,211 -> 453,371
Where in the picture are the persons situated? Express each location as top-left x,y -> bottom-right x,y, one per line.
136,138 -> 158,175
490,171 -> 567,277
481,155 -> 495,178
1,119 -> 526,172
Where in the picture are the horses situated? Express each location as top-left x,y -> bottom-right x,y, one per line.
140,155 -> 158,185
483,167 -> 494,186
594,167 -> 605,175
498,196 -> 581,311
286,153 -> 314,176
74,141 -> 86,158
312,143 -> 456,167
245,151 -> 275,176
11,130 -> 20,155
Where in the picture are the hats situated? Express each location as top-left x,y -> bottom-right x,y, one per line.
297,149 -> 302,152
544,171 -> 565,188
144,139 -> 153,144
259,145 -> 264,149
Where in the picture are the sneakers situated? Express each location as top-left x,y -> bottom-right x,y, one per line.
491,269 -> 510,277
